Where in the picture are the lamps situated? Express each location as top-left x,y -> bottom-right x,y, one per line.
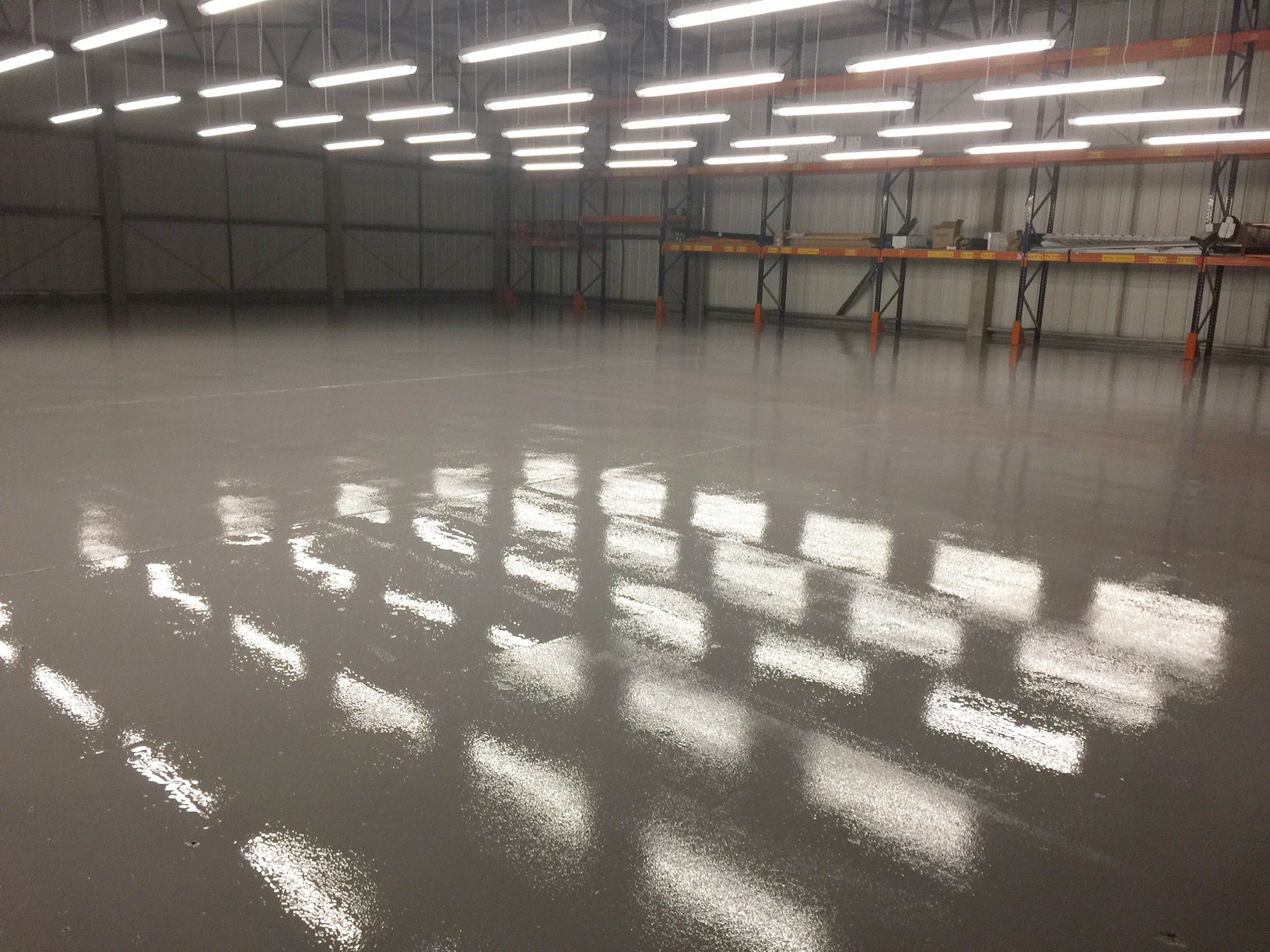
2,0 -> 1270,180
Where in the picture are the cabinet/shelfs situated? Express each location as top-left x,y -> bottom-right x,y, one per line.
494,2 -> 1270,361
